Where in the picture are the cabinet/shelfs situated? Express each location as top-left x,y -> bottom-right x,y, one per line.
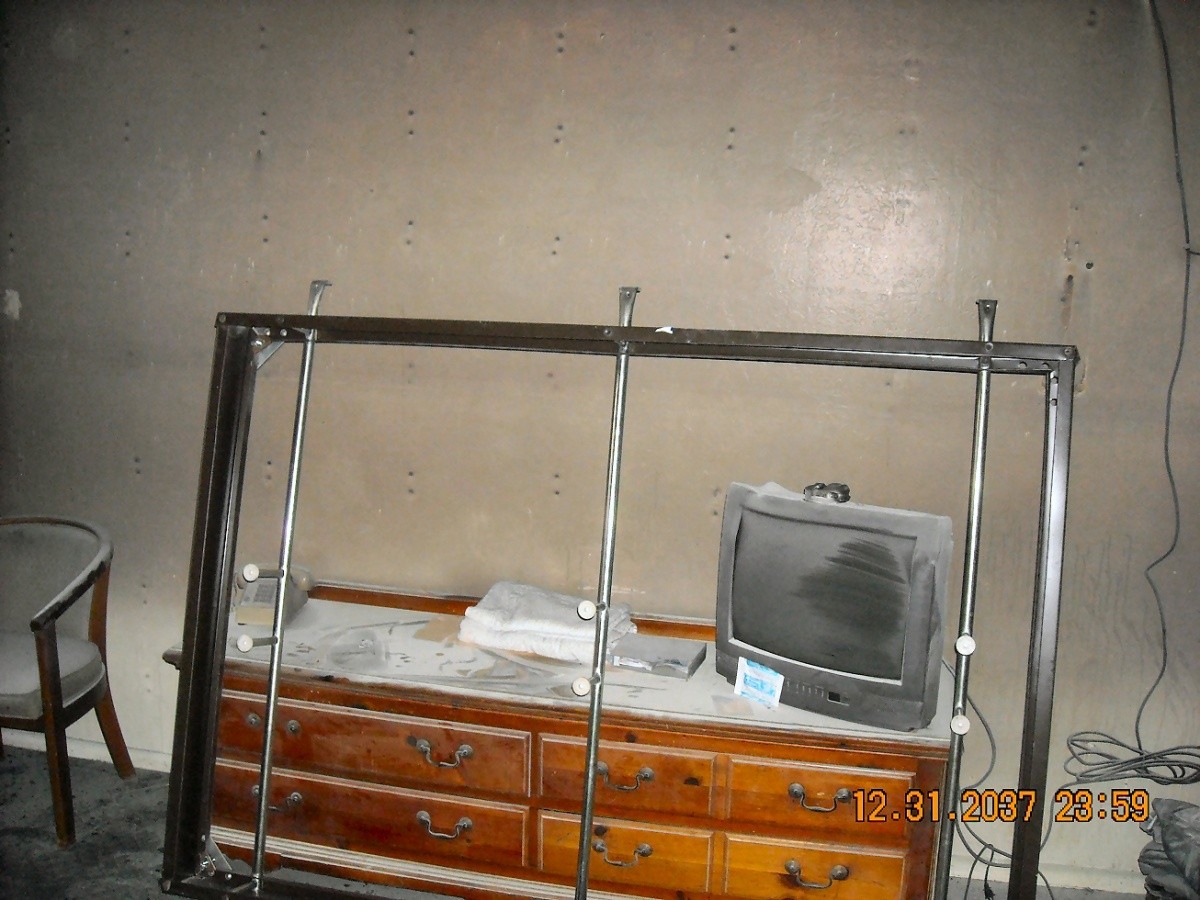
162,572 -> 966,900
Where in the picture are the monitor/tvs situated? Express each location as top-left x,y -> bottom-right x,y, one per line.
715,482 -> 953,733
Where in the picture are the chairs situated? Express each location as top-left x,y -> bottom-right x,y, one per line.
0,514 -> 133,847
